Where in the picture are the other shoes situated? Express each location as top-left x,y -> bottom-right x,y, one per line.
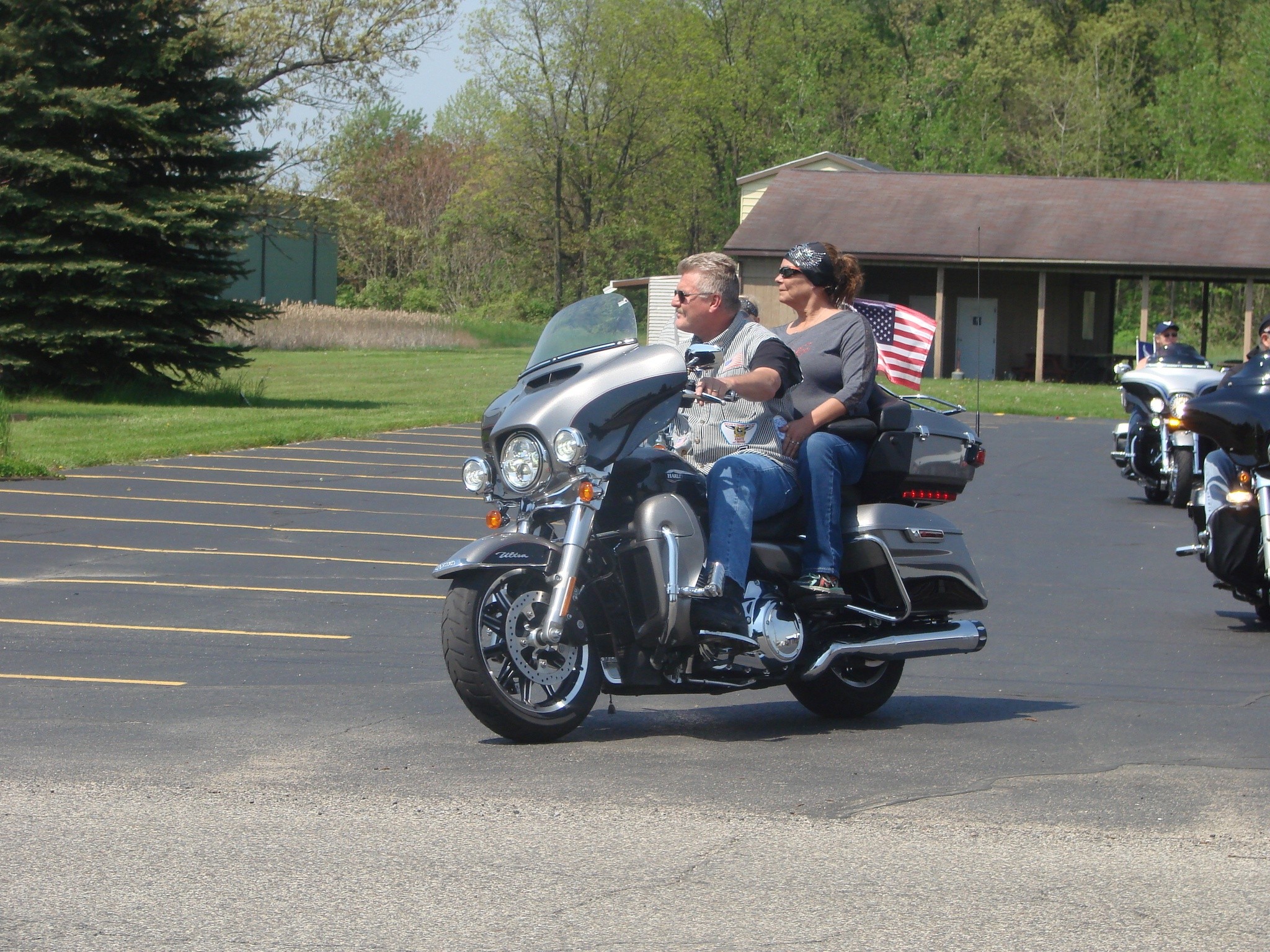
1120,465 -> 1138,481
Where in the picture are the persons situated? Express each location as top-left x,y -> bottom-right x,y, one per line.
1204,311 -> 1270,555
763,240 -> 880,594
1124,321 -> 1180,475
670,251 -> 807,638
733,290 -> 762,328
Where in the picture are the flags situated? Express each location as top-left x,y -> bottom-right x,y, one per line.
833,297 -> 939,392
1136,338 -> 1153,368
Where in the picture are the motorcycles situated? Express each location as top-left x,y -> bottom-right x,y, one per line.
1176,351 -> 1270,626
433,292 -> 990,743
1111,342 -> 1230,509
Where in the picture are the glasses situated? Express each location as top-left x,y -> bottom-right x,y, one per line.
1164,332 -> 1178,338
674,290 -> 714,304
778,267 -> 801,279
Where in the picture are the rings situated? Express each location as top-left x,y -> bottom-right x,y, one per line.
712,389 -> 719,393
785,425 -> 789,428
790,440 -> 796,445
796,441 -> 801,445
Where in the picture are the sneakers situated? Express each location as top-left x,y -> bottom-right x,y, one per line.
790,569 -> 845,594
692,581 -> 748,638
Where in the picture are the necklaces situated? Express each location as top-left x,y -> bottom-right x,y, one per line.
795,303 -> 831,334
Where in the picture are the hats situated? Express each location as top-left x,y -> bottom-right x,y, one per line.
1155,321 -> 1179,333
1258,315 -> 1270,336
